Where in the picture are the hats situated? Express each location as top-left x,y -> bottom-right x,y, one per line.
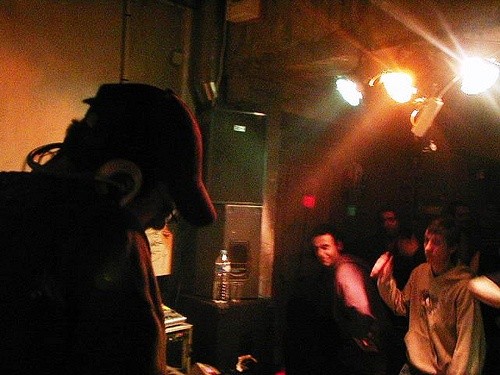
83,81 -> 216,229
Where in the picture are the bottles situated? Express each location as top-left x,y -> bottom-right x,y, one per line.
213,250 -> 231,303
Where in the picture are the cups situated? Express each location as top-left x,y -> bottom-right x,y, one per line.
230,282 -> 244,303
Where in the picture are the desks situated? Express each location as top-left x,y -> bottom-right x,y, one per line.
180,293 -> 285,375
166,321 -> 193,374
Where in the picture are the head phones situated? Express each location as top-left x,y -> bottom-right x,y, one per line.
27,143 -> 143,209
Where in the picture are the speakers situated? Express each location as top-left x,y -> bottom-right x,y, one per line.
180,203 -> 272,300
194,104 -> 268,206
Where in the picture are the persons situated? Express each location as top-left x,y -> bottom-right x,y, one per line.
375,207 -> 425,375
398,205 -> 480,289
308,224 -> 394,375
0,83 -> 217,375
468,270 -> 500,309
370,216 -> 487,375
